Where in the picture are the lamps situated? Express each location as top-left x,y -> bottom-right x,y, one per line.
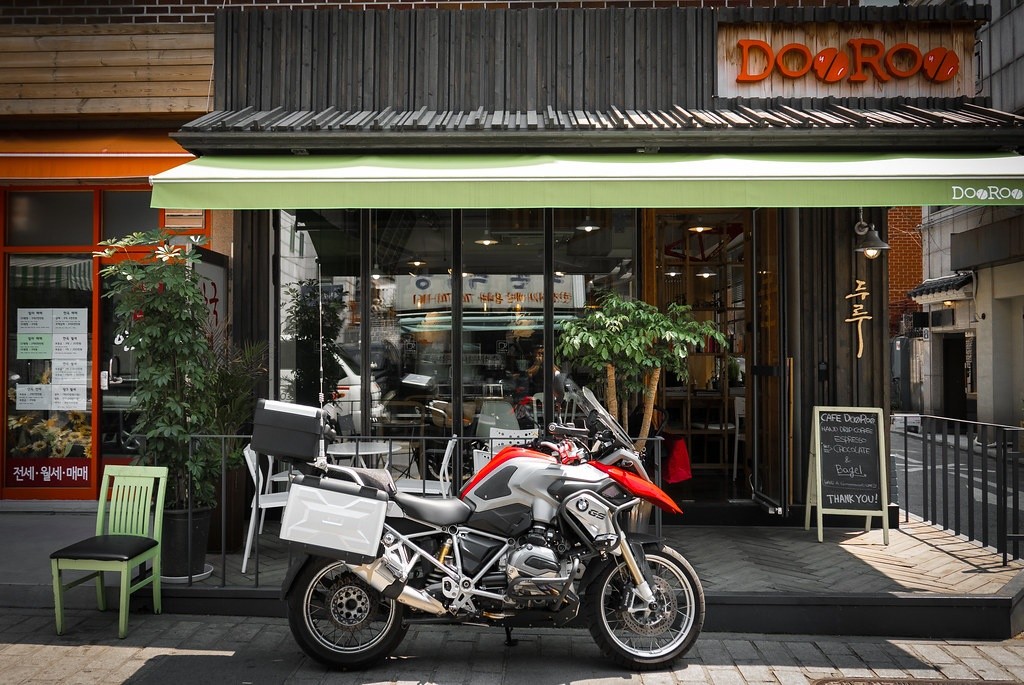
406,254 -> 427,266
575,216 -> 601,232
370,210 -> 387,279
854,221 -> 891,260
474,209 -> 499,246
688,216 -> 713,233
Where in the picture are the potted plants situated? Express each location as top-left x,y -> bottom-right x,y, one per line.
198,311 -> 275,555
93,225 -> 244,582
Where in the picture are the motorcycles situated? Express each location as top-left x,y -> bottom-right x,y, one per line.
400,372 -> 569,483
252,369 -> 706,671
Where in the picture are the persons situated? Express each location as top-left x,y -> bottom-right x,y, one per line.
514,380 -> 530,395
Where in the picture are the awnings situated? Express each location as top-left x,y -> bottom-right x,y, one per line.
145,147 -> 1023,210
0,134 -> 200,188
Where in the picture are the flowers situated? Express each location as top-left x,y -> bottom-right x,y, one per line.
7,368 -> 92,458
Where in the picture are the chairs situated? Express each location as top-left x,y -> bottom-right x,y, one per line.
395,433 -> 458,500
488,427 -> 539,453
531,391 -> 576,434
473,449 -> 498,474
259,455 -> 290,535
732,396 -> 746,480
241,443 -> 290,574
49,464 -> 169,639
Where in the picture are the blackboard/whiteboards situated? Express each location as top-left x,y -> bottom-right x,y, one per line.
806,405 -> 888,517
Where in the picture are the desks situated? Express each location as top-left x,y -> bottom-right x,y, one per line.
326,442 -> 402,468
